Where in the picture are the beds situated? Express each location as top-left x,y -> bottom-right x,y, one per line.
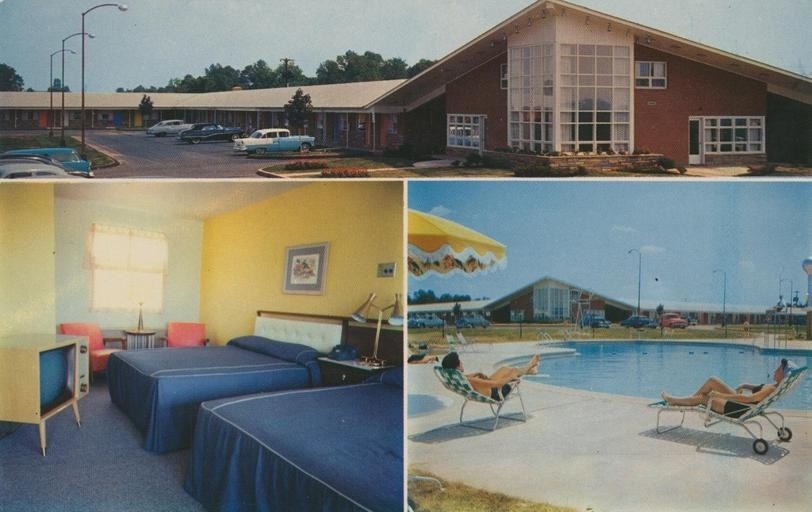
106,308 -> 346,455
182,364 -> 403,512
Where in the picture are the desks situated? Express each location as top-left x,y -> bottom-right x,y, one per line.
122,327 -> 156,349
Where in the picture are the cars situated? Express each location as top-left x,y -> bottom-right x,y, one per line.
233,128 -> 316,156
620,315 -> 657,329
408,313 -> 446,329
454,314 -> 489,329
579,313 -> 610,329
146,119 -> 195,138
653,312 -> 687,329
177,122 -> 242,144
680,316 -> 697,326
0,148 -> 95,180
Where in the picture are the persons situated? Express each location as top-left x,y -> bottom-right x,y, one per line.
743,321 -> 749,334
409,352 -> 439,364
442,351 -> 541,401
409,342 -> 450,349
659,357 -> 800,419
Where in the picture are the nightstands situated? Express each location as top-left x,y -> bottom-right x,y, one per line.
318,356 -> 394,387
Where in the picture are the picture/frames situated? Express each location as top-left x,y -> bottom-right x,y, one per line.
282,241 -> 330,297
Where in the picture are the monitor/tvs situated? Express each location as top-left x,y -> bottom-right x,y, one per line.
0,332 -> 90,424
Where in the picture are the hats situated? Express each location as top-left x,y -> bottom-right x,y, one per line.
784,360 -> 800,379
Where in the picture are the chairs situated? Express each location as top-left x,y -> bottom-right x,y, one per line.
158,321 -> 210,348
432,364 -> 528,432
408,327 -> 479,369
60,322 -> 128,386
647,362 -> 812,456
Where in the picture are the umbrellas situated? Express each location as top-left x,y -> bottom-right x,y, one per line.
406,206 -> 509,284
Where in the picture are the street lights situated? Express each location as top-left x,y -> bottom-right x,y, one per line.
780,277 -> 792,327
49,50 -> 76,140
628,248 -> 641,316
81,3 -> 129,162
59,32 -> 95,149
713,268 -> 725,327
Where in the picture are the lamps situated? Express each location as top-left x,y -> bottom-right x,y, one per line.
349,290 -> 404,368
